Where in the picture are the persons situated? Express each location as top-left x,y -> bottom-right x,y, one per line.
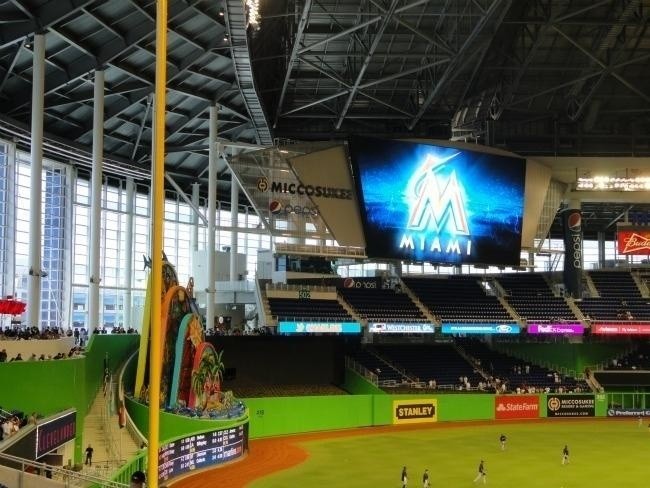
608,351 -> 650,370
372,356 -> 587,396
499,433 -> 508,449
84,442 -> 94,466
103,366 -> 112,399
0,404 -> 40,442
130,470 -> 148,488
473,459 -> 487,484
421,468 -> 430,488
0,323 -> 141,364
616,297 -> 634,320
560,444 -> 571,464
401,466 -> 410,488
204,325 -> 266,336
